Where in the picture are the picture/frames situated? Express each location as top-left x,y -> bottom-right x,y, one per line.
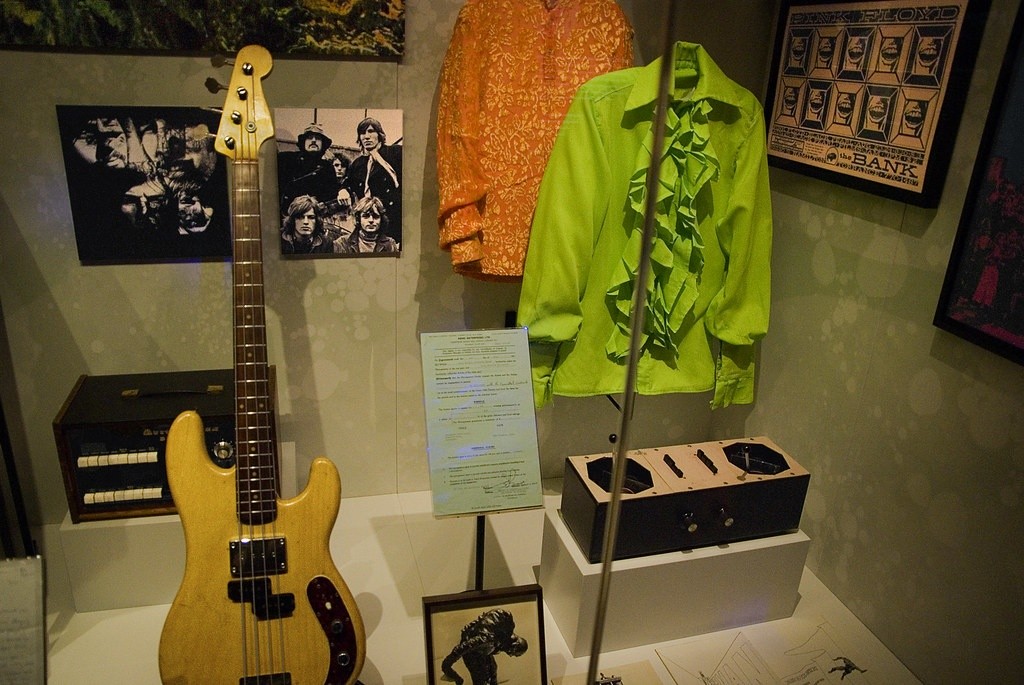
932,0 -> 1024,368
420,584 -> 548,685
765,0 -> 993,210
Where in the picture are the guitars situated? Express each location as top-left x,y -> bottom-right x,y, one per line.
155,43 -> 366,685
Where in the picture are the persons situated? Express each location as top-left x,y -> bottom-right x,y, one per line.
279,117 -> 399,254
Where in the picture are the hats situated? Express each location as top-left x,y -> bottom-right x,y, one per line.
296,125 -> 332,152
